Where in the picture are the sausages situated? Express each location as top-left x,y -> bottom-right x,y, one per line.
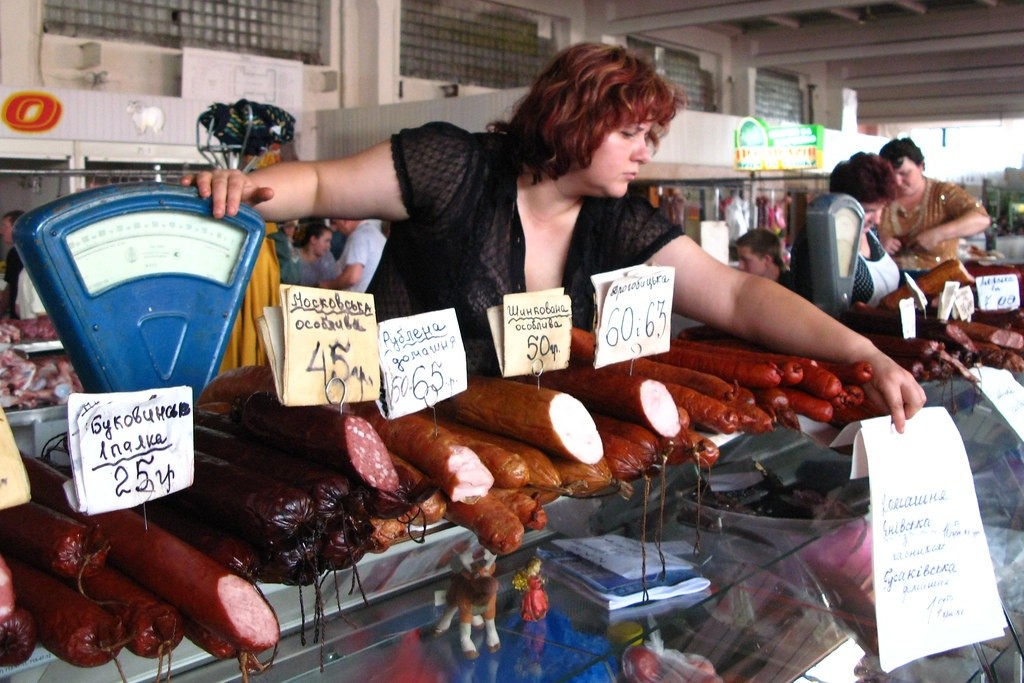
2,260 -> 1024,666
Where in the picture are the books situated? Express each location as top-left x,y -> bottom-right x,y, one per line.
532,546 -> 712,611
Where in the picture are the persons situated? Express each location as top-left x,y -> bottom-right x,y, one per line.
1,207 -> 27,320
266,218 -> 389,292
14,267 -> 47,320
791,150 -> 903,308
873,137 -> 993,271
512,556 -> 550,622
181,33 -> 929,434
994,218 -> 1024,237
735,229 -> 794,290
